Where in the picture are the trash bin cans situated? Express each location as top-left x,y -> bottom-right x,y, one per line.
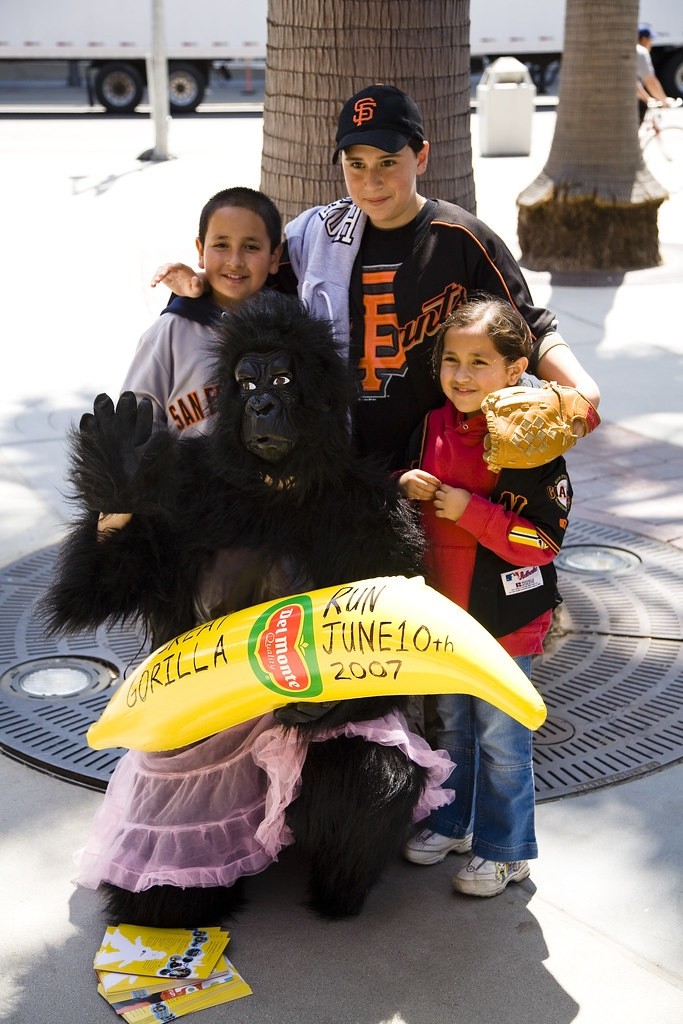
476,57 -> 538,158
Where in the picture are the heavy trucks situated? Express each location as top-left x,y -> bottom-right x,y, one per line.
0,0 -> 683,116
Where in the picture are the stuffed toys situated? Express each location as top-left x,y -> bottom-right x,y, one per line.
47,290 -> 459,929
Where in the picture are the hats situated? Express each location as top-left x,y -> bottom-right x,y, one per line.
332,81 -> 424,165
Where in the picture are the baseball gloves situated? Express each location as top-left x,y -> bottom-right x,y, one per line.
480,384 -> 602,472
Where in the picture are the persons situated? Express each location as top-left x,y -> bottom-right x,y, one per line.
114,186 -> 286,439
383,296 -> 573,897
150,85 -> 600,468
635,25 -> 675,135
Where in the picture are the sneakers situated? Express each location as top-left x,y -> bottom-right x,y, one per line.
403,826 -> 474,865
452,856 -> 531,897
